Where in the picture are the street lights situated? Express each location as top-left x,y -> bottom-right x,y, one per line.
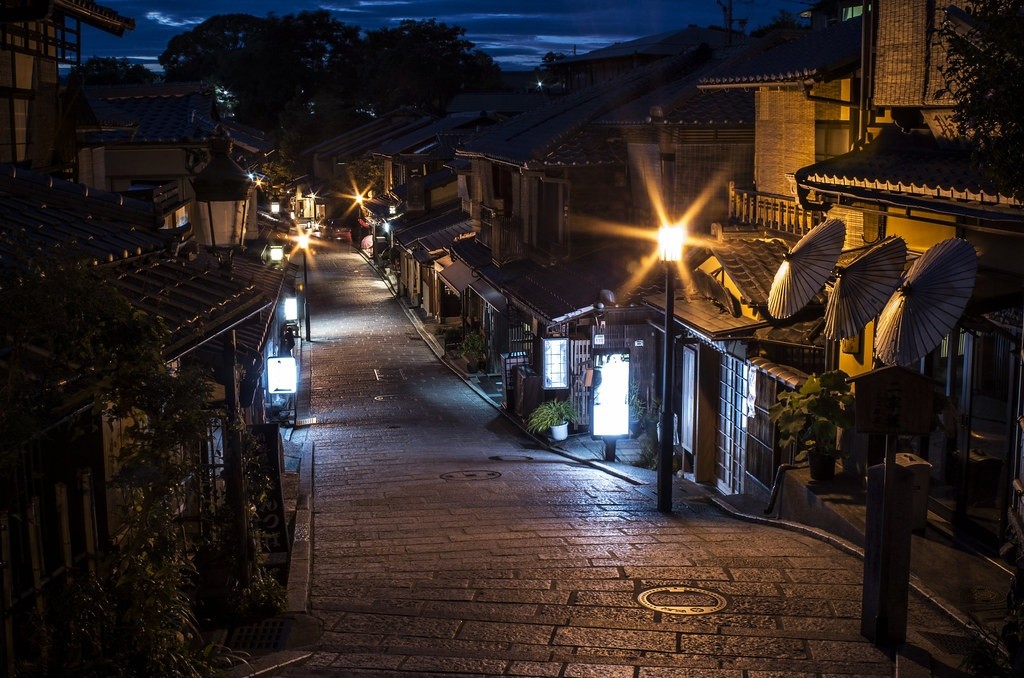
297,233 -> 311,341
655,223 -> 686,509
356,195 -> 363,250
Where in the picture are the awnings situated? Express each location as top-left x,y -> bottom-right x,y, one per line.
468,278 -> 510,314
438,258 -> 482,298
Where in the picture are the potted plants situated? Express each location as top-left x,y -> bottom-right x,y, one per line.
527,398 -> 579,441
768,368 -> 855,482
462,330 -> 486,374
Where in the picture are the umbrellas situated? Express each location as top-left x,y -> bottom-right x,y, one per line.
767,217 -> 847,320
823,234 -> 909,341
874,236 -> 981,367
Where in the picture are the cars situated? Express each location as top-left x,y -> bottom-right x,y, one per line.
323,217 -> 354,241
